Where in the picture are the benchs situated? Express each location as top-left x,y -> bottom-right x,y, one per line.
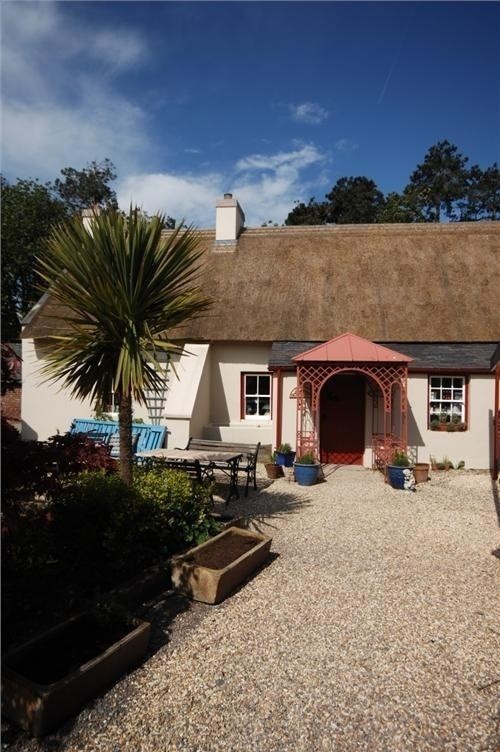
168,437 -> 260,497
133,455 -> 215,508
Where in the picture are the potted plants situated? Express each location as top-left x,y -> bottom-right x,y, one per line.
431,413 -> 466,432
386,449 -> 416,489
264,443 -> 321,486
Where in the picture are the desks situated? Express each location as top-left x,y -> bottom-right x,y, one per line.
135,448 -> 243,507
